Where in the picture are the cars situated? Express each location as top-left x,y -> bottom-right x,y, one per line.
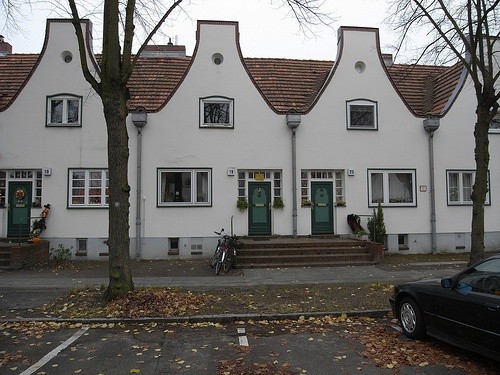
389,254 -> 500,361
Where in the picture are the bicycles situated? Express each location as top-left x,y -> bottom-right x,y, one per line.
210,229 -> 238,275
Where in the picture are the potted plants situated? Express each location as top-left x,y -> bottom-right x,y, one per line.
367,203 -> 387,258
28,220 -> 38,243
